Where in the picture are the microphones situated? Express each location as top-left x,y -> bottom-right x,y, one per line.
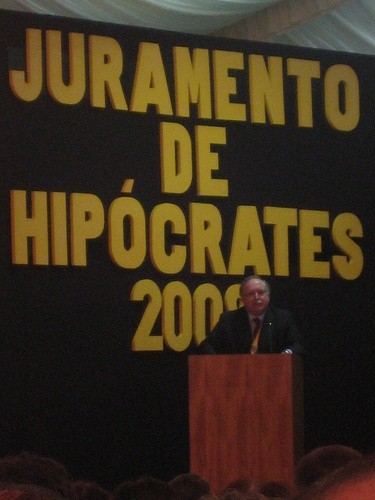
267,323 -> 272,353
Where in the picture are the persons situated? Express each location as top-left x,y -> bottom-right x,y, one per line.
0,444 -> 375,500
199,274 -> 303,355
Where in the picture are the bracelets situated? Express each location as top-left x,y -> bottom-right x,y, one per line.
284,350 -> 290,353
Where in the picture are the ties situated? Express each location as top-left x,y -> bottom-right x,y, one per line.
250,318 -> 262,355
